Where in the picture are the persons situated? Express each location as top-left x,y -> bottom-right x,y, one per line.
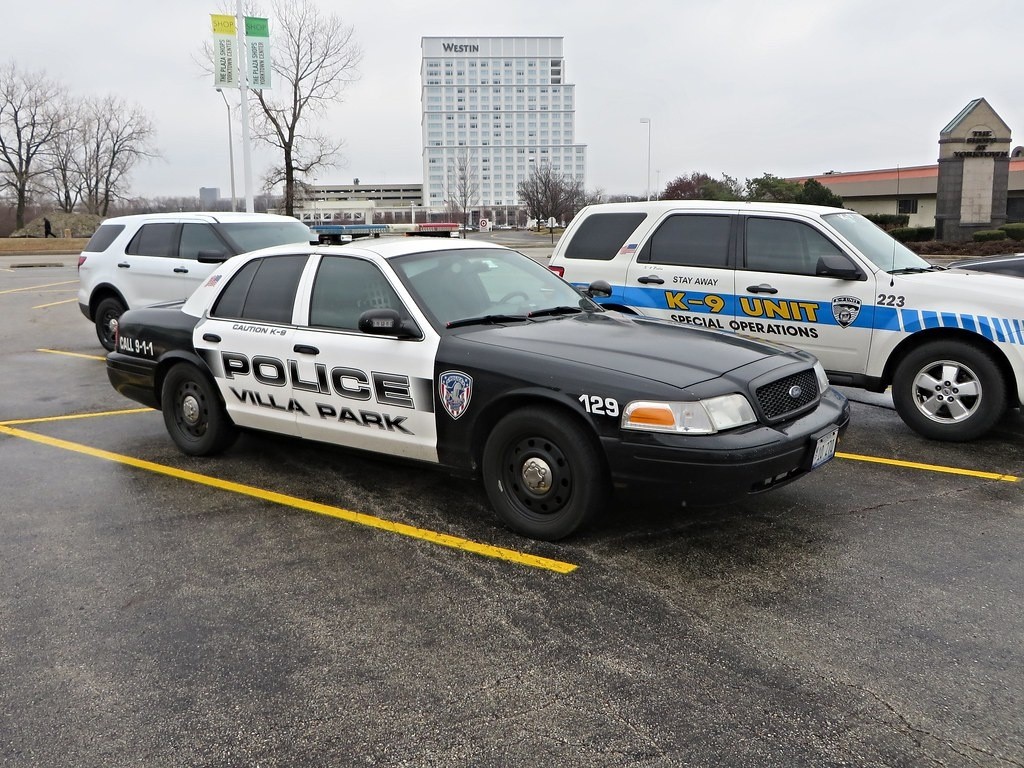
44,217 -> 57,238
429,262 -> 522,324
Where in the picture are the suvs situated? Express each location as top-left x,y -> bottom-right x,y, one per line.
536,198 -> 1024,444
74,210 -> 446,353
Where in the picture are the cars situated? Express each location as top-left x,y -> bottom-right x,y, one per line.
946,253 -> 1024,278
101,222 -> 852,545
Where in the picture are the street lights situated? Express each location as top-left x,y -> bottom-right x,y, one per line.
216,87 -> 238,212
639,118 -> 651,202
